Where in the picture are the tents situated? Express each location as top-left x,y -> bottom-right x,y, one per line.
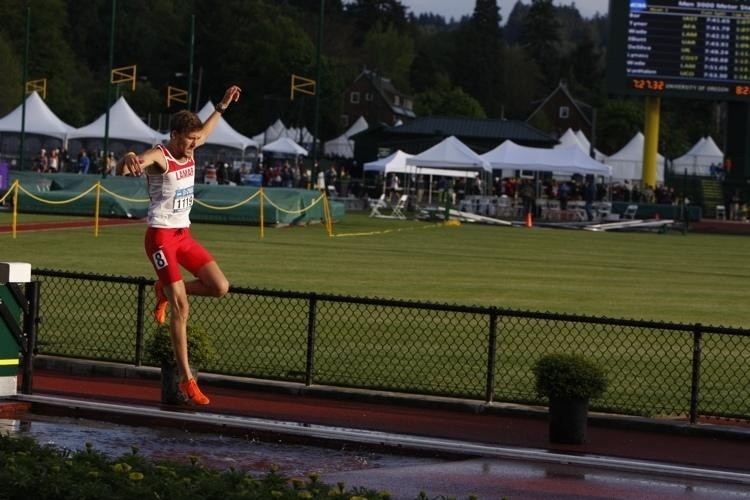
364,136 -> 726,203
261,137 -> 308,177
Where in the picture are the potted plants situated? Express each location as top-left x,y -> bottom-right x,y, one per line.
145,322 -> 218,405
532,352 -> 607,444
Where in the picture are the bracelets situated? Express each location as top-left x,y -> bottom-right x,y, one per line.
124,151 -> 135,159
215,102 -> 226,114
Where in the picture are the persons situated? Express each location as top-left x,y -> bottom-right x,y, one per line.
203,157 -> 345,196
115,84 -> 243,407
1,160 -> 14,208
36,146 -> 118,193
363,173 -> 749,228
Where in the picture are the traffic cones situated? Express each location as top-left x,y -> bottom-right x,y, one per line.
524,212 -> 534,230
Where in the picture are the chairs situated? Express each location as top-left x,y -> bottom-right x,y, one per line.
327,182 -> 749,221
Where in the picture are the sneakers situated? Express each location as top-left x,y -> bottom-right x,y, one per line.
153,280 -> 170,324
178,378 -> 210,407
